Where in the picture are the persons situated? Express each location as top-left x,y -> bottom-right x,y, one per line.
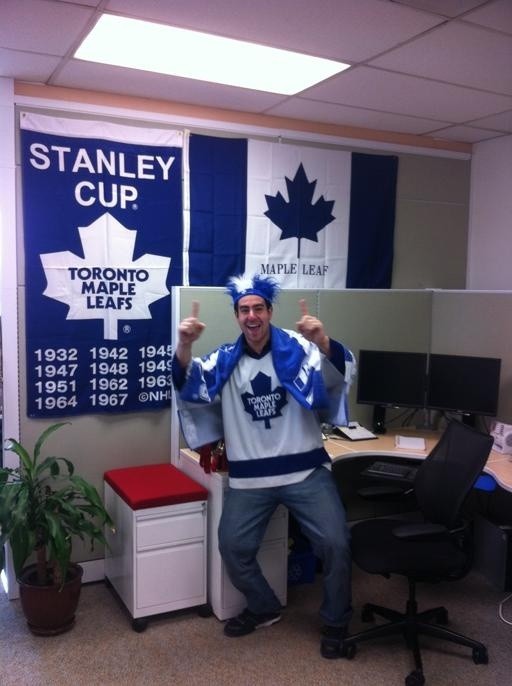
169,274 -> 359,658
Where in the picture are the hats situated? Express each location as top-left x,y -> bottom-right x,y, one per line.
224,273 -> 281,306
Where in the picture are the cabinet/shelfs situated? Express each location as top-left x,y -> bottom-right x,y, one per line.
103,474 -> 207,619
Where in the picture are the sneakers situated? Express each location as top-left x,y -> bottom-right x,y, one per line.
319,625 -> 348,658
223,607 -> 282,638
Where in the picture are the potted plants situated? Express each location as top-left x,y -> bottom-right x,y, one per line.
1,419 -> 116,637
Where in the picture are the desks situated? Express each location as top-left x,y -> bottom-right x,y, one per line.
180,422 -> 511,624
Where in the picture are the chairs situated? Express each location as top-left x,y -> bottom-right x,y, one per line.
337,419 -> 496,686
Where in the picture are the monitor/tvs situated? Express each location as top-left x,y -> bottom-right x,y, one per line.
425,351 -> 502,432
355,349 -> 426,435
